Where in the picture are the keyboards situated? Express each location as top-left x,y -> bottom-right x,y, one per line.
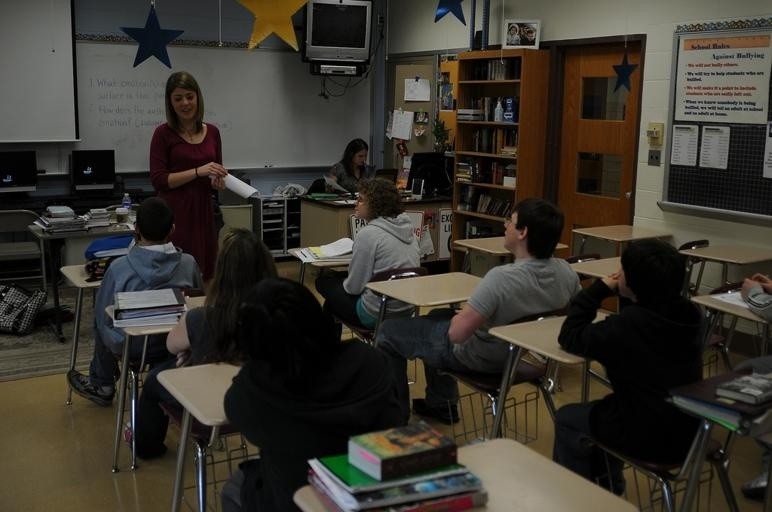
0,186 -> 36,193
76,184 -> 113,190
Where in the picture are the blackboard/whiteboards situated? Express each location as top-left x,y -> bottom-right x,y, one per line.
656,24 -> 772,228
32,32 -> 374,177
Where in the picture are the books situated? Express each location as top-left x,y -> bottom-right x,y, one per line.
307,419 -> 488,512
671,370 -> 772,428
32,205 -> 111,236
294,238 -> 355,262
439,60 -> 518,258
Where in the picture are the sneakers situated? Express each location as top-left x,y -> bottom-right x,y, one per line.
67,370 -> 115,407
124,422 -> 133,442
412,398 -> 460,424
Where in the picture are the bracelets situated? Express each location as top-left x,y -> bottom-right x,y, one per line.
194,166 -> 201,179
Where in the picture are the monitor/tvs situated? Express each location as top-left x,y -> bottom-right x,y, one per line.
306,0 -> 372,60
405,152 -> 445,199
71,149 -> 116,185
0,149 -> 38,188
375,167 -> 398,185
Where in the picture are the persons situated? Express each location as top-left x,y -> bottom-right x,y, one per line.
734,272 -> 772,499
375,197 -> 580,424
220,274 -> 405,512
325,140 -> 370,194
554,238 -> 702,496
507,24 -> 520,46
124,227 -> 288,459
66,196 -> 204,407
520,29 -> 535,45
149,70 -> 227,281
316,176 -> 420,342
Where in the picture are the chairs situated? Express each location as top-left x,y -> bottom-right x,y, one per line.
1,210 -> 52,292
440,313 -> 563,448
592,368 -> 752,509
678,239 -> 709,301
112,332 -> 170,469
187,424 -> 251,510
332,266 -> 427,388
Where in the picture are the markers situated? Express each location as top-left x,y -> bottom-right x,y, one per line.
265,165 -> 271,167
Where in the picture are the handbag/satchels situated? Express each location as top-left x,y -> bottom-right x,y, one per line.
0,285 -> 47,335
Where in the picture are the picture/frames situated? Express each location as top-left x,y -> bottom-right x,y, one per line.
503,19 -> 542,50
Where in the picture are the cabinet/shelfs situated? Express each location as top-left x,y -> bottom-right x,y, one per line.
216,194 -> 300,266
450,48 -> 550,275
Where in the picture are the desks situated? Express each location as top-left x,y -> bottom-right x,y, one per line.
288,249 -> 351,281
666,365 -> 771,506
363,270 -> 486,345
690,291 -> 769,362
680,242 -> 772,347
455,234 -> 567,270
295,191 -> 451,267
571,257 -> 630,316
105,296 -> 208,472
292,438 -> 640,512
58,262 -> 104,407
486,312 -> 614,443
571,225 -> 672,261
155,360 -> 240,510
28,212 -> 137,343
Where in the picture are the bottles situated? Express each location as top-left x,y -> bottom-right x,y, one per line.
494,101 -> 504,122
122,193 -> 132,212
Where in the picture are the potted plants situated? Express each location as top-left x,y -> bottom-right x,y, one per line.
432,117 -> 452,152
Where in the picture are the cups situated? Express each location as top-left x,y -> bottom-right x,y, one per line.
115,208 -> 129,226
